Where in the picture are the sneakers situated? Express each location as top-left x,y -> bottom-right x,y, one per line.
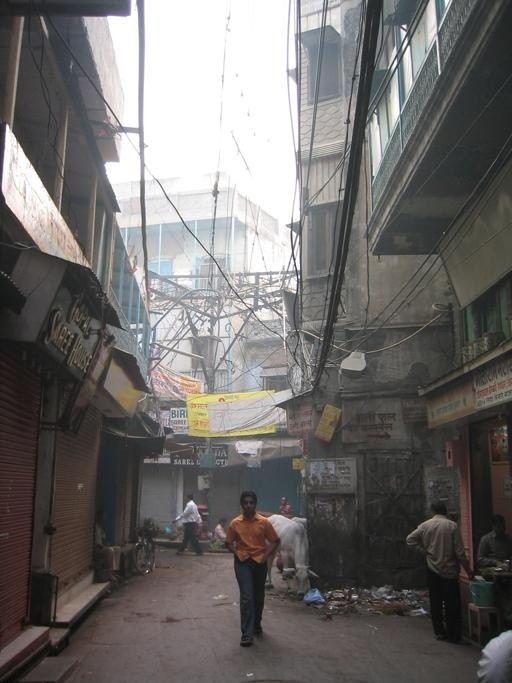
240,623 -> 264,647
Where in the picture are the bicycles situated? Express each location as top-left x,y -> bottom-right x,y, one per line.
133,526 -> 155,575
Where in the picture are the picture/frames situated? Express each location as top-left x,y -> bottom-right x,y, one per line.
489,428 -> 508,465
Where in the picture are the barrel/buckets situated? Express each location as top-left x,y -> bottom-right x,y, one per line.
467,579 -> 493,607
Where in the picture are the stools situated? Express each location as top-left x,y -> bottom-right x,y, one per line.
468,604 -> 501,643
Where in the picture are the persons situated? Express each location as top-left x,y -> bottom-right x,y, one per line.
213,518 -> 226,546
406,500 -> 474,645
279,496 -> 293,519
476,515 -> 512,568
476,629 -> 512,683
225,491 -> 281,647
171,493 -> 203,555
94,511 -> 120,582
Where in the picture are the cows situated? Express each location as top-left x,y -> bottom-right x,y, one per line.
257,512 -> 319,596
